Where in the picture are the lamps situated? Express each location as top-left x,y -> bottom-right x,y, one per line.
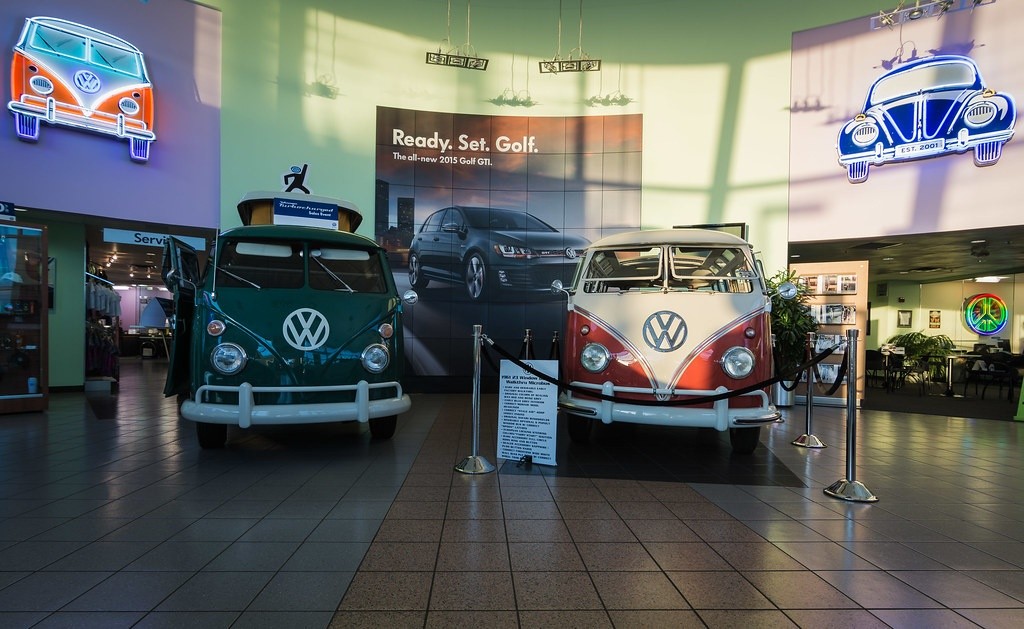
539,0 -> 601,71
870,0 -> 996,30
425,0 -> 489,69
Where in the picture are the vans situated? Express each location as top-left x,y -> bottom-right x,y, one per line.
162,191 -> 420,447
550,228 -> 798,431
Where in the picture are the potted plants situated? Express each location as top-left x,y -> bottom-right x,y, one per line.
762,271 -> 820,407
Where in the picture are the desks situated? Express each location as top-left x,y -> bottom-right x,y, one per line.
919,354 -> 982,397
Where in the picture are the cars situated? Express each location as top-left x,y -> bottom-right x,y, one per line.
408,206 -> 594,301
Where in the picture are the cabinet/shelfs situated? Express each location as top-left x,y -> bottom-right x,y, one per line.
0,218 -> 49,412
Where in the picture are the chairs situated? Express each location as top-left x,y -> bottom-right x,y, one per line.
865,349 -> 1024,402
